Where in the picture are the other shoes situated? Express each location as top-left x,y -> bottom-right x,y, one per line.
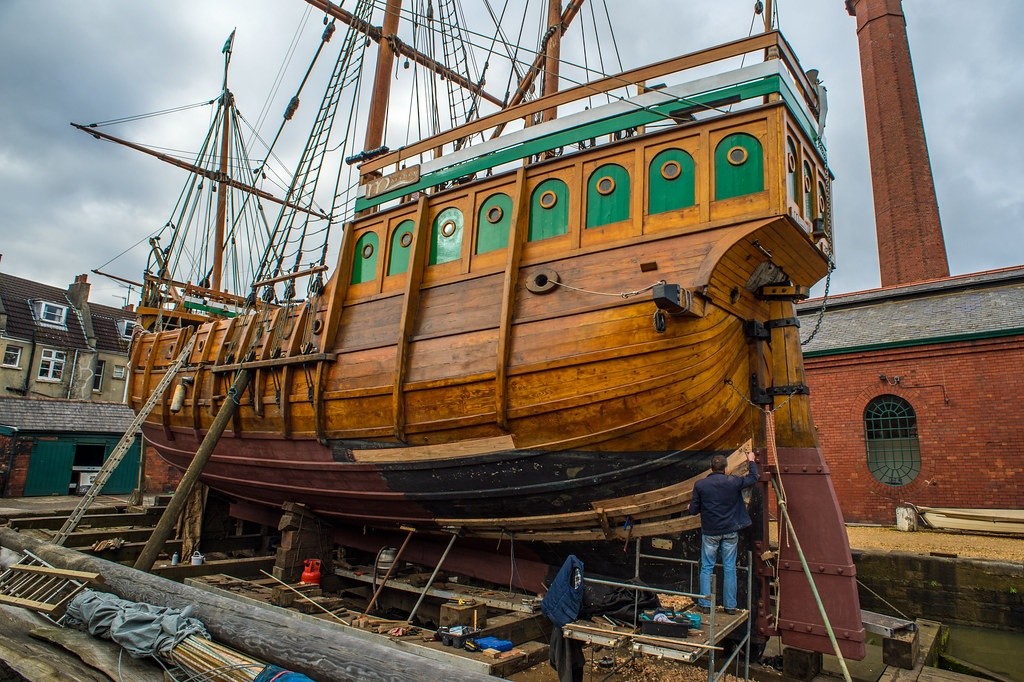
697,605 -> 710,613
724,607 -> 736,614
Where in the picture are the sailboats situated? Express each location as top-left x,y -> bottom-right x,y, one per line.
64,3 -> 838,597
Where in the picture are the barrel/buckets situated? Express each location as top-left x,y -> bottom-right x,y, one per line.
191,551 -> 203,565
896,507 -> 918,532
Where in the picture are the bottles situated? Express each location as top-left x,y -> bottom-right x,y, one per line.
172,552 -> 178,565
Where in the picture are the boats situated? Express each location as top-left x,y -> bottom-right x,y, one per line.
904,499 -> 1024,536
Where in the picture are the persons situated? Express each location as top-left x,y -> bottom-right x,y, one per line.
687,451 -> 758,615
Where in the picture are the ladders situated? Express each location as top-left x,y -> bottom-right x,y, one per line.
52,334 -> 198,546
0,548 -> 106,630
